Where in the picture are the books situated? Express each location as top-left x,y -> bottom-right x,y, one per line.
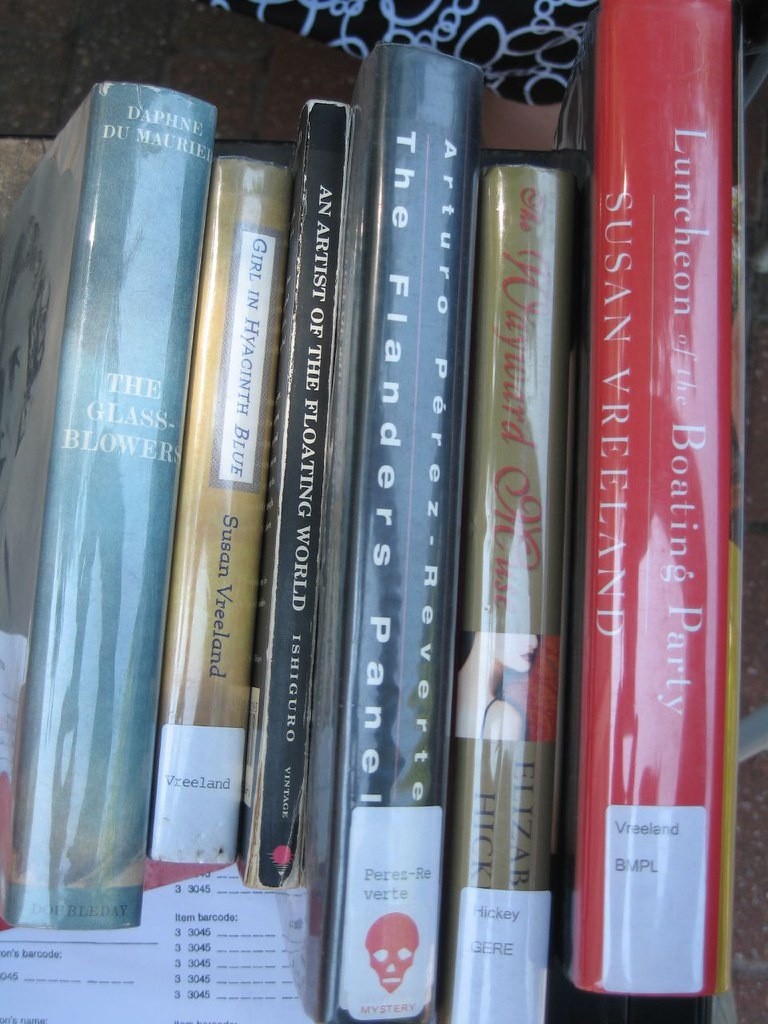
1,0 -> 736,1024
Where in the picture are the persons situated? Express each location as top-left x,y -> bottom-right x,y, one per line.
455,629 -> 538,741
